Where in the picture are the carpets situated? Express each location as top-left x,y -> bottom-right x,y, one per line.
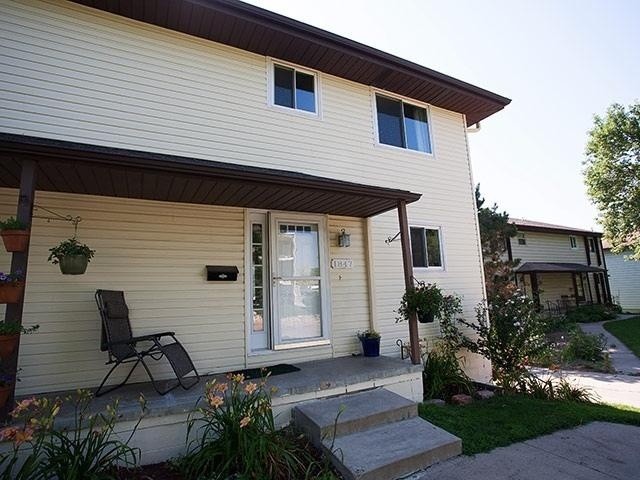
226,363 -> 301,381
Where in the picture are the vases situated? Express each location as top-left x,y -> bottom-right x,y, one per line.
0,286 -> 24,303
417,310 -> 434,323
0,387 -> 10,407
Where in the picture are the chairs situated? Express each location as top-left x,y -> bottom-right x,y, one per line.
93,289 -> 201,396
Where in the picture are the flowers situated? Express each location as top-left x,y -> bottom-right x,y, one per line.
0,265 -> 26,287
0,361 -> 23,387
393,283 -> 463,324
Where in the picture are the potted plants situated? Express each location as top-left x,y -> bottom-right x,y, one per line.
0,318 -> 40,358
0,216 -> 30,252
356,329 -> 381,357
48,239 -> 96,275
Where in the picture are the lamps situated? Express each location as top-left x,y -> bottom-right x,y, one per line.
338,228 -> 350,247
